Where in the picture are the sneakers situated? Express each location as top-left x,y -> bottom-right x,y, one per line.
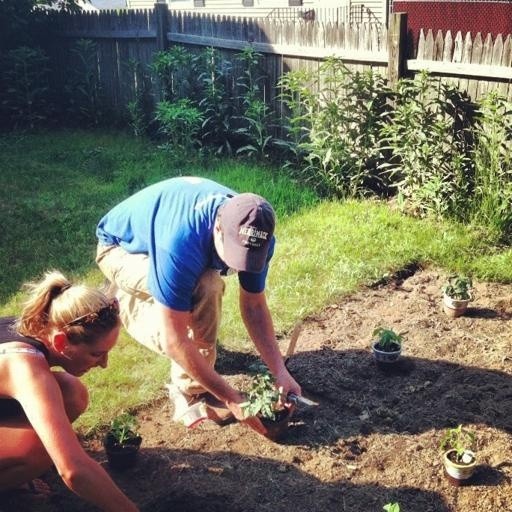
169,377 -> 209,429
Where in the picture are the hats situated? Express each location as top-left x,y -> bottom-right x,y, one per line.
220,192 -> 275,273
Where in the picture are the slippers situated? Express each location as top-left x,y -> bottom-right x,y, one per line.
11,478 -> 43,495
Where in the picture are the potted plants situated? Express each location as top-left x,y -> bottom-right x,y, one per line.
101,413 -> 143,467
372,326 -> 402,363
444,273 -> 472,319
241,371 -> 291,440
439,424 -> 477,480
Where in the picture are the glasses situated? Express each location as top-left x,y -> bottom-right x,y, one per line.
66,297 -> 120,326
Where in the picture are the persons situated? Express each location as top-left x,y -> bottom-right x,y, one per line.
96,174 -> 300,434
1,270 -> 141,511
